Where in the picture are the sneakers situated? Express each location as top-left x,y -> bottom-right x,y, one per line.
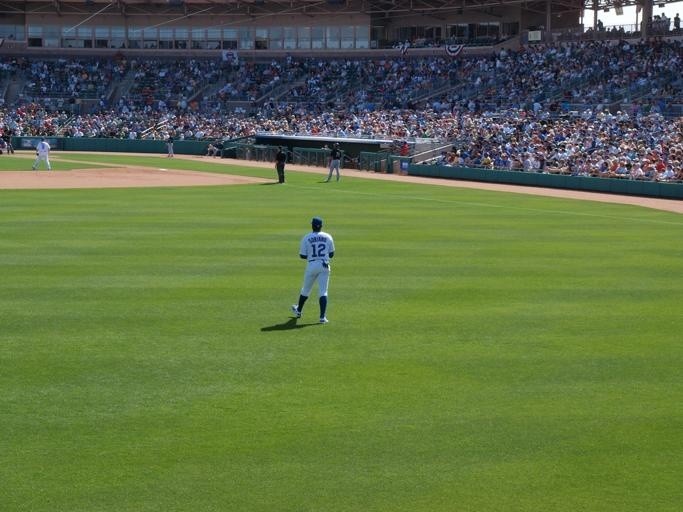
320,317 -> 328,323
291,305 -> 301,316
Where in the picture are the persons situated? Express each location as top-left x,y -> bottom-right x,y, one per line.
289,217 -> 335,324
1,12 -> 681,185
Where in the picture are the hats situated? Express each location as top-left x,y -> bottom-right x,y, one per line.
312,217 -> 322,227
